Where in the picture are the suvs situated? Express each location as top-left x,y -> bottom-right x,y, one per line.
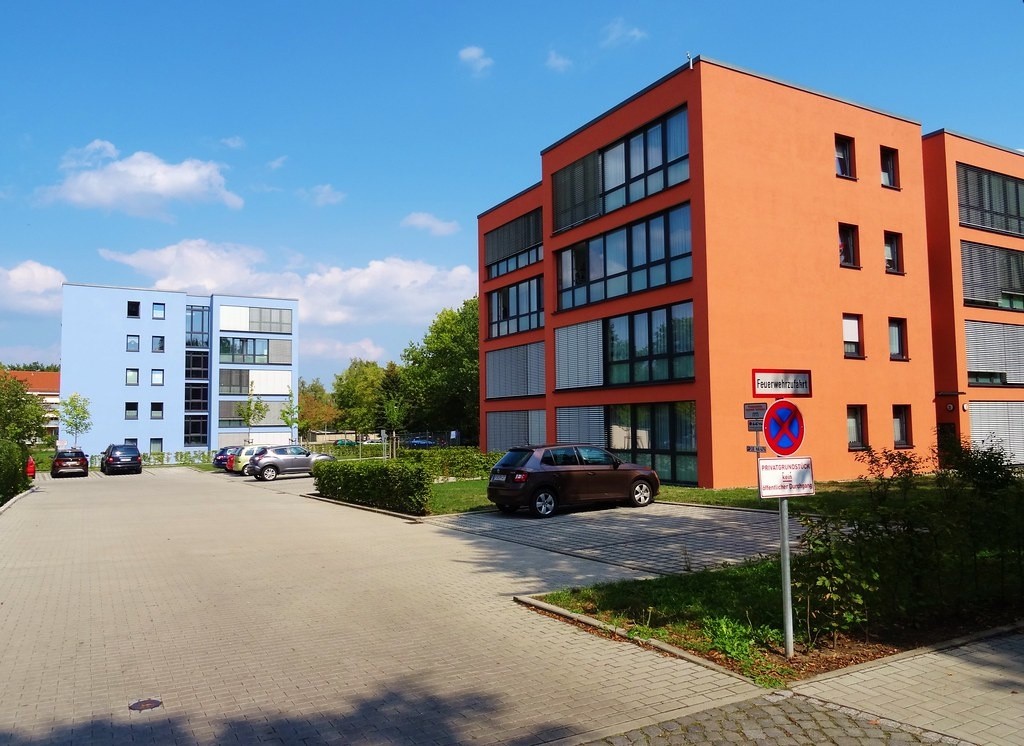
247,446 -> 337,481
100,444 -> 142,474
486,443 -> 661,517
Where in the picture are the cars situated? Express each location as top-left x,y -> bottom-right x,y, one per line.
410,436 -> 435,444
333,439 -> 357,446
49,450 -> 89,478
26,456 -> 35,479
213,446 -> 267,476
363,439 -> 389,444
